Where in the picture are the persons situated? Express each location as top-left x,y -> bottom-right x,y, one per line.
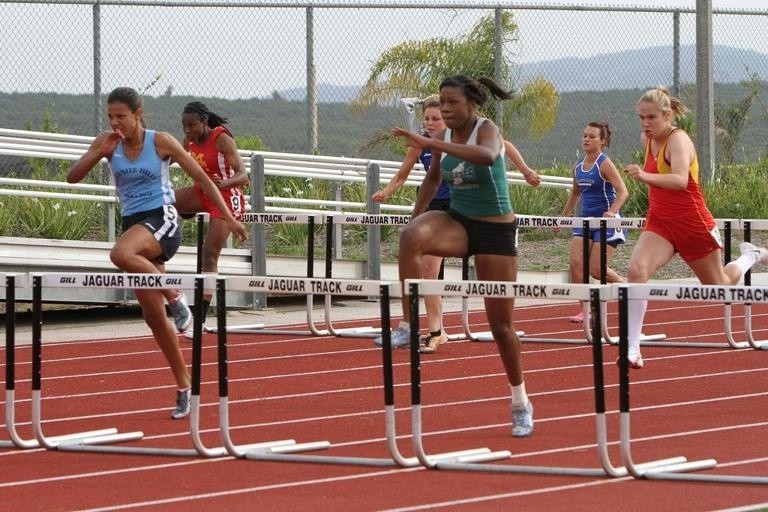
371,94 -> 542,354
552,120 -> 629,325
616,84 -> 768,370
374,74 -> 534,438
169,101 -> 248,341
66,87 -> 247,420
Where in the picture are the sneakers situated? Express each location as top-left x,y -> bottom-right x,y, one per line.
615,351 -> 644,369
570,311 -> 591,323
171,386 -> 191,419
374,327 -> 411,349
184,322 -> 205,339
169,291 -> 192,333
418,329 -> 447,353
510,400 -> 534,436
739,242 -> 768,267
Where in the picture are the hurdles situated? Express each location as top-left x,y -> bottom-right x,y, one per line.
0,213 -> 767,483
